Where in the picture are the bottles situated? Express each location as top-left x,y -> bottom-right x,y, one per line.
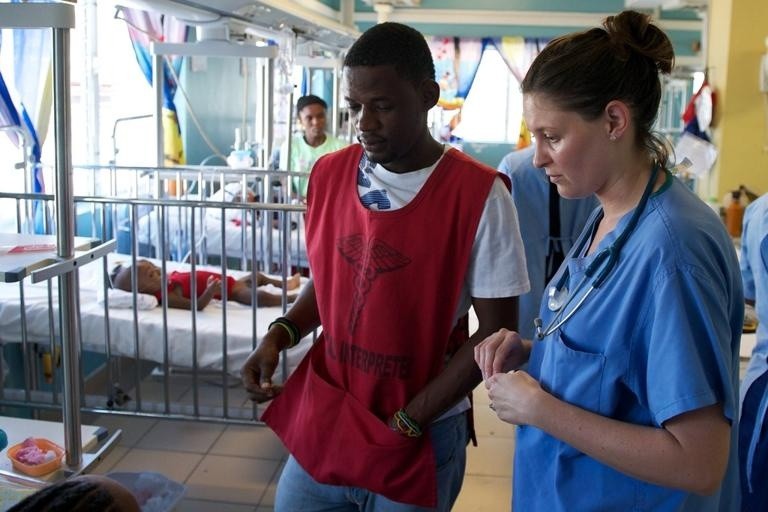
723,189 -> 744,236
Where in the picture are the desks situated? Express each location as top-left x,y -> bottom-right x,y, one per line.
0,412 -> 123,489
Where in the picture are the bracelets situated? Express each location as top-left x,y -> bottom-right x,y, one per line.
267,318 -> 302,350
393,409 -> 424,440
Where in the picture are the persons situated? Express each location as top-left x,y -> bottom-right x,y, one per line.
112,258 -> 304,309
499,141 -> 601,375
232,193 -> 301,229
280,96 -> 359,204
8,475 -> 140,510
238,20 -> 531,512
737,189 -> 768,512
471,8 -> 746,512
